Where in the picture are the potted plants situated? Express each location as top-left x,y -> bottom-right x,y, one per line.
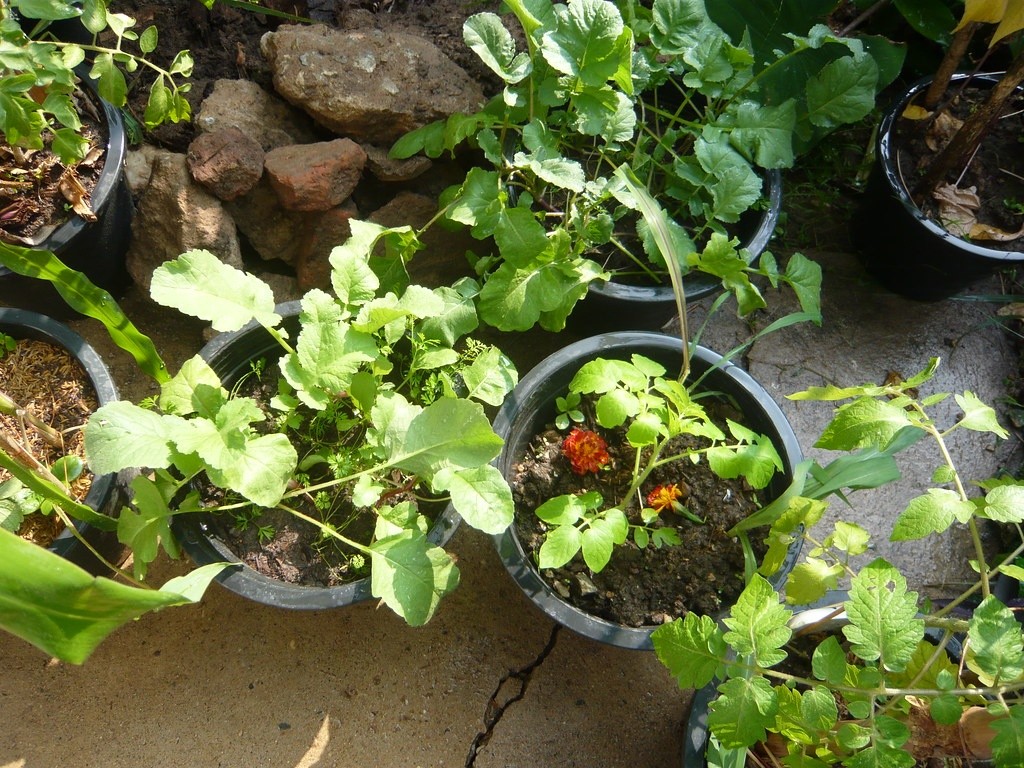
0,0 -> 1024,768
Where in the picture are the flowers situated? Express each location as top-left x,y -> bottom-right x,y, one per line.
647,483 -> 707,525
563,427 -> 610,474
